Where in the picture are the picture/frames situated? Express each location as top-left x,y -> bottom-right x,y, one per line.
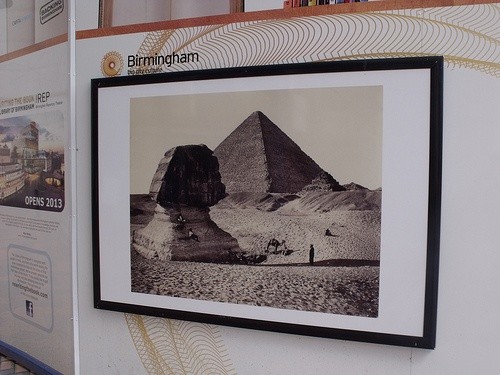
89,54 -> 445,350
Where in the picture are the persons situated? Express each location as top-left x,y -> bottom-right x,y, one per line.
176,213 -> 201,243
281,240 -> 288,257
309,244 -> 314,266
